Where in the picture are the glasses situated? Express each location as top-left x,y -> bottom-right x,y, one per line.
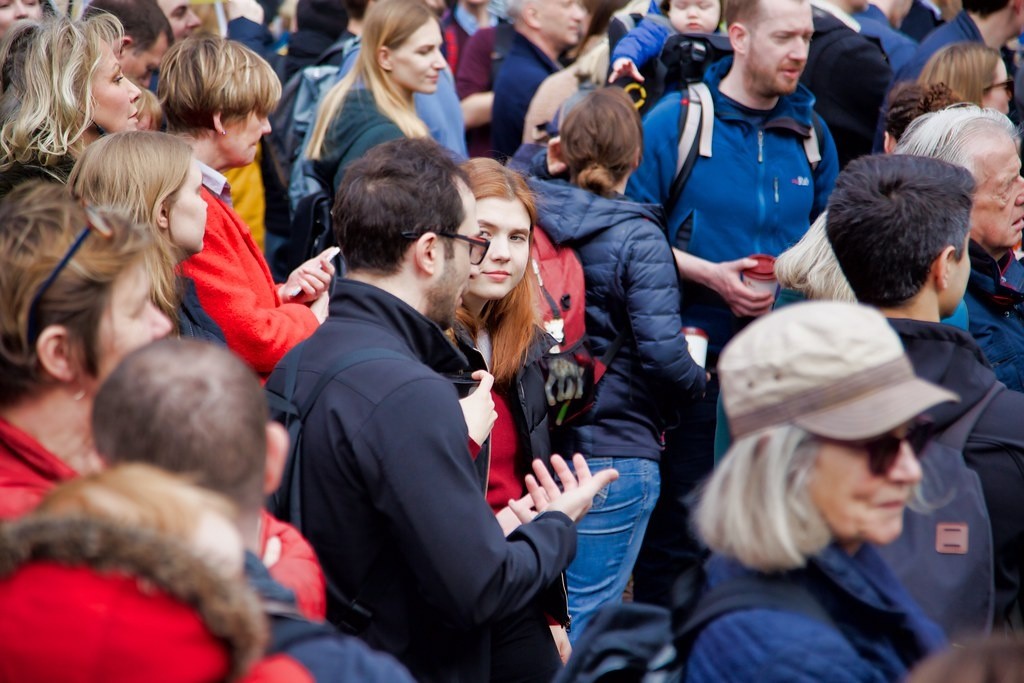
815,418 -> 936,474
403,231 -> 490,265
985,75 -> 1014,99
28,197 -> 114,346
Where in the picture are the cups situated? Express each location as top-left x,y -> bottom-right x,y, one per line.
743,255 -> 779,311
682,326 -> 708,370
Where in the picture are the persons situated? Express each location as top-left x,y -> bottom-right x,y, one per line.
265,137 -> 619,683
0,0 -> 1024,683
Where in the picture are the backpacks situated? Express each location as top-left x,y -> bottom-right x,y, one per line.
273,159 -> 334,278
261,38 -> 361,237
866,379 -> 1007,640
551,578 -> 846,683
260,337 -> 413,632
528,223 -> 628,432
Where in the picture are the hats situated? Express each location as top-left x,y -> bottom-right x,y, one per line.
716,301 -> 961,440
546,91 -> 591,137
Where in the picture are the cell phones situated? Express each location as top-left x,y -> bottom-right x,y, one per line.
288,247 -> 340,296
